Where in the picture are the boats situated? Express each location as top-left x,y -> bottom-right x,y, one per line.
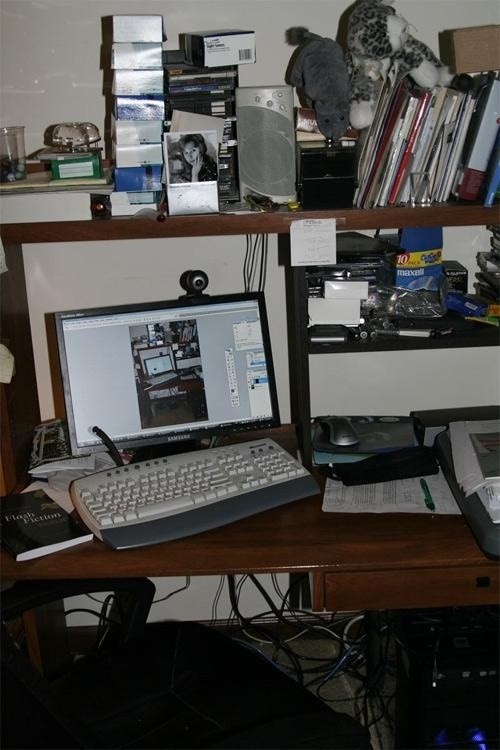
235,85 -> 297,206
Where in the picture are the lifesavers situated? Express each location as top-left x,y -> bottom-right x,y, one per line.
395,606 -> 500,750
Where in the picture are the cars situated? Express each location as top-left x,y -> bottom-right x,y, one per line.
410,171 -> 430,207
0,125 -> 26,181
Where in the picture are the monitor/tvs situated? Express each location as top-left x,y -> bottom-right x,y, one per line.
355,68 -> 499,208
474,224 -> 500,302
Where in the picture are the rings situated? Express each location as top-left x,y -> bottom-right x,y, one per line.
420,478 -> 435,510
347,317 -> 377,341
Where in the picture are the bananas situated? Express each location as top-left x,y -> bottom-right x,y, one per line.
69,436 -> 321,551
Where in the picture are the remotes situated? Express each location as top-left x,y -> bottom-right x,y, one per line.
319,416 -> 361,446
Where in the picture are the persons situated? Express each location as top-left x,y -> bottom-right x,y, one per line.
178,134 -> 217,181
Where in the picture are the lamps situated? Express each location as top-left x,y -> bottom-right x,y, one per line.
0,577 -> 374,750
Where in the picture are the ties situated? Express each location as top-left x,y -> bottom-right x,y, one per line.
54,291 -> 281,466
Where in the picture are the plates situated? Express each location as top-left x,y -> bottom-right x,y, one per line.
0,204 -> 500,678
